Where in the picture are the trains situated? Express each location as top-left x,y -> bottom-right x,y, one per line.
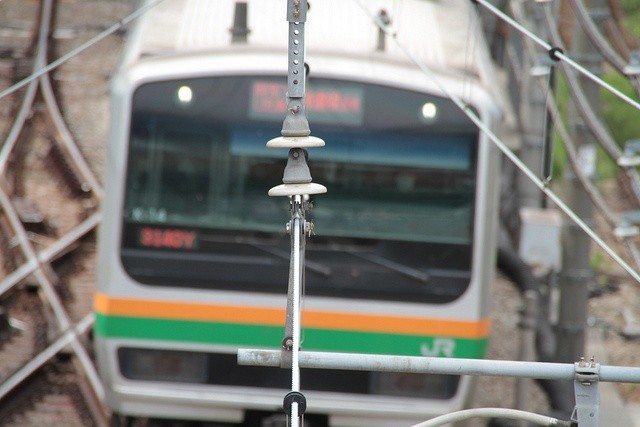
91,0 -> 504,427
